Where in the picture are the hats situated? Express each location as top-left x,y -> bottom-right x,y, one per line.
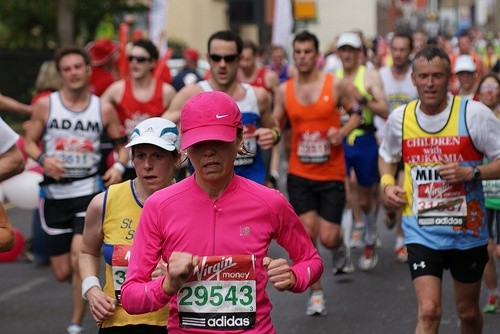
336,31 -> 363,48
180,91 -> 244,151
86,38 -> 120,66
453,54 -> 477,76
182,47 -> 202,60
124,117 -> 183,155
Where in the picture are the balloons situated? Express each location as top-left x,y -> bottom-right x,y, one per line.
0,129 -> 45,211
0,228 -> 23,263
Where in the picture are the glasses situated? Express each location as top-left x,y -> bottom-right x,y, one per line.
209,53 -> 239,61
128,55 -> 152,62
480,83 -> 499,92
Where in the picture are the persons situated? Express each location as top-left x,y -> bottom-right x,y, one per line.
100,39 -> 174,167
374,30 -> 420,264
82,37 -> 122,97
120,87 -> 324,334
268,45 -> 297,83
24,48 -> 103,334
239,39 -> 281,89
0,96 -> 34,118
0,117 -> 27,183
319,26 -> 500,115
272,31 -> 364,318
169,47 -> 205,91
0,200 -> 15,254
377,46 -> 500,334
159,31 -> 274,185
473,74 -> 500,314
32,56 -> 62,101
77,114 -> 183,334
334,30 -> 384,272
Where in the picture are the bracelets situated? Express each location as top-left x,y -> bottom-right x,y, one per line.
359,96 -> 369,106
80,275 -> 101,301
377,174 -> 395,194
272,129 -> 283,145
36,152 -> 49,167
113,162 -> 126,177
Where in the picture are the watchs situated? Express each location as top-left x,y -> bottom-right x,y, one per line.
473,165 -> 485,185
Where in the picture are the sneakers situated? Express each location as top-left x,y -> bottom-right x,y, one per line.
482,290 -> 499,313
394,236 -> 408,262
385,211 -> 398,229
359,233 -> 378,270
364,226 -> 382,248
305,289 -> 327,315
350,222 -> 365,248
67,324 -> 82,334
332,243 -> 355,274
